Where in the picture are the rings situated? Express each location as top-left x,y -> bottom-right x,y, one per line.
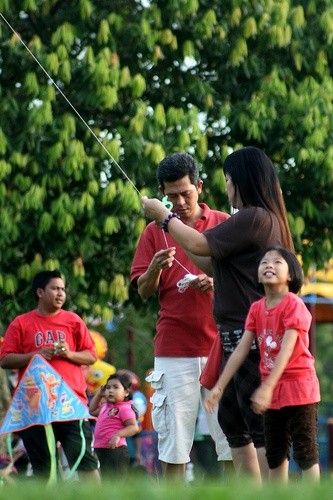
61,347 -> 66,352
200,282 -> 203,286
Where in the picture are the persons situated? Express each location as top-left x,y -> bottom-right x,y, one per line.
0,146 -> 321,483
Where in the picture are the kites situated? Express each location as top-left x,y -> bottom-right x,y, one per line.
0,353 -> 97,489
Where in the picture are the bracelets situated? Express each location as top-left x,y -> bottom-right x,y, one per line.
162,213 -> 180,233
